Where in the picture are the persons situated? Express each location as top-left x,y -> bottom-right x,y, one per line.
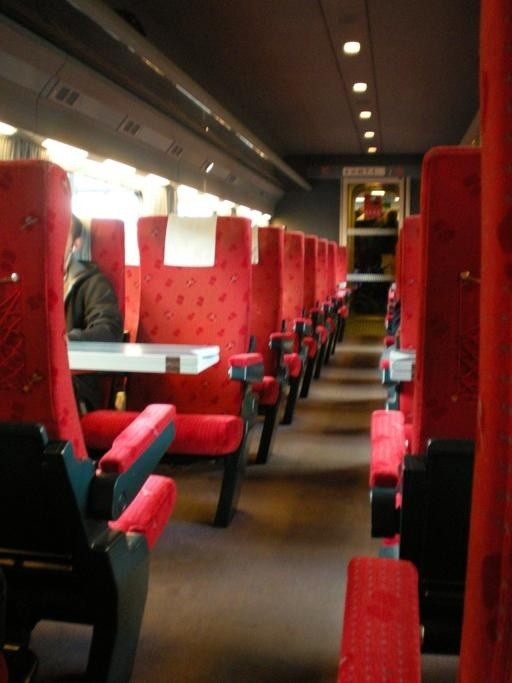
58,212 -> 123,418
376,208 -> 399,254
389,297 -> 401,342
